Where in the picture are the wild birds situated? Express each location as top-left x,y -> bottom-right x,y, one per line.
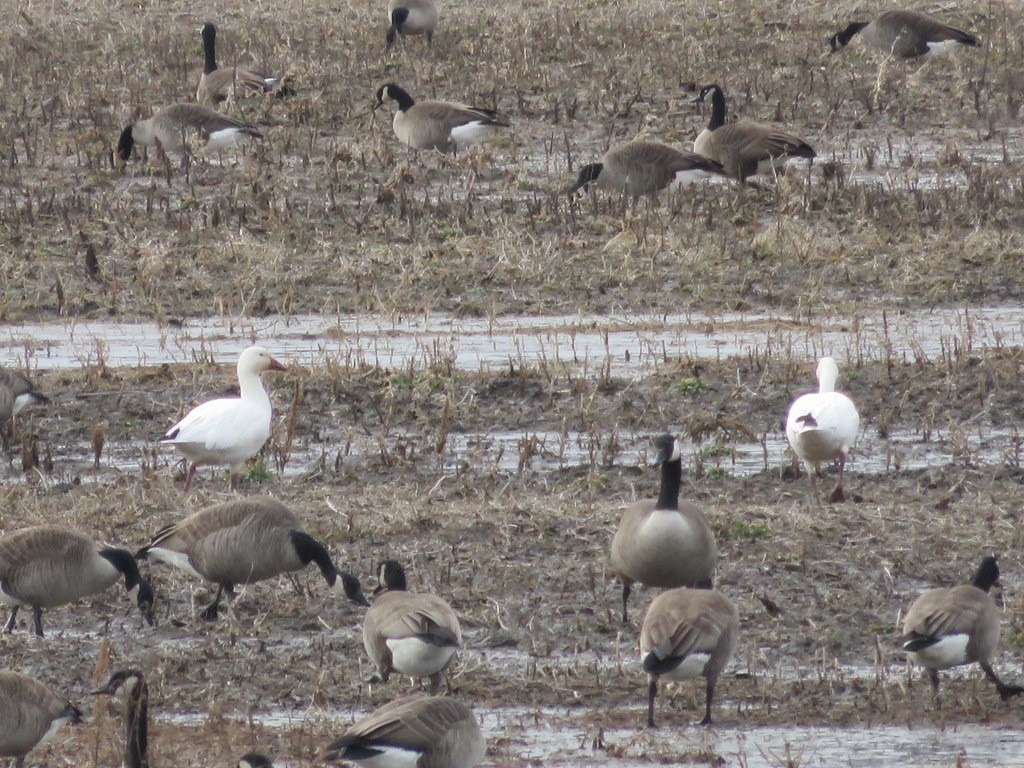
197,20 -> 299,129
119,101 -> 265,191
612,434 -> 742,729
827,6 -> 984,82
0,365 -> 50,453
901,556 -> 1024,711
786,356 -> 861,508
1,522 -> 155,637
687,82 -> 817,212
383,0 -> 437,55
1,667 -> 486,767
369,80 -> 512,173
562,138 -> 728,217
362,560 -> 464,697
132,493 -> 375,627
158,346 -> 288,498
